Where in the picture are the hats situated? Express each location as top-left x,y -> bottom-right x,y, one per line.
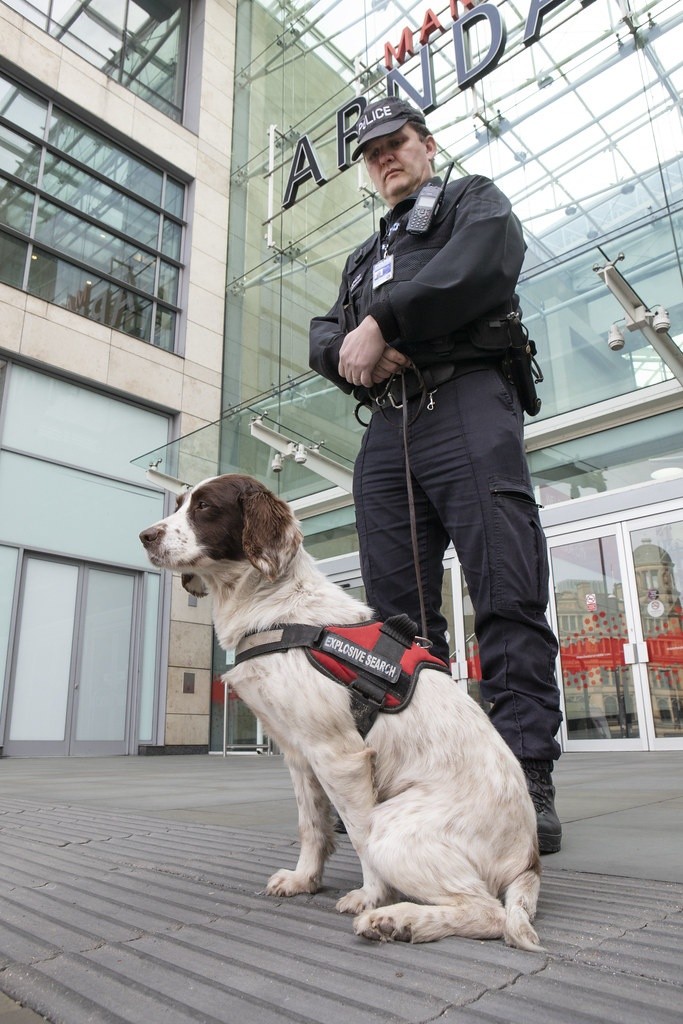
351,97 -> 426,161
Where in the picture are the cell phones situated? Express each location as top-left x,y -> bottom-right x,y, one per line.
405,162 -> 455,236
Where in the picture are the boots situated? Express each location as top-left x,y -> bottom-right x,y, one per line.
524,767 -> 562,852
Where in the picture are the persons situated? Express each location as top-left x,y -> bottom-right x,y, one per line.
306,98 -> 563,856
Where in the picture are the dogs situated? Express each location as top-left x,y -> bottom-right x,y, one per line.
139,473 -> 551,954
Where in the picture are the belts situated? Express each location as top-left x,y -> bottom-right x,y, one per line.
371,357 -> 500,414
